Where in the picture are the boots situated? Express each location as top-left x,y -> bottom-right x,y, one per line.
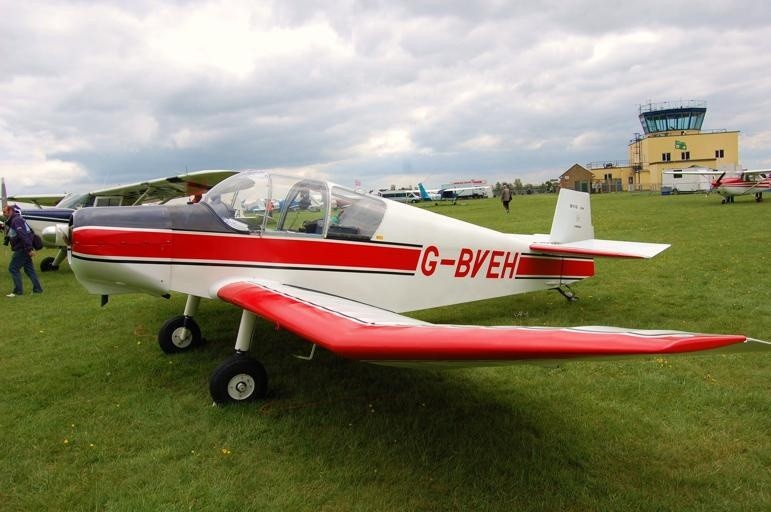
6,293 -> 21,297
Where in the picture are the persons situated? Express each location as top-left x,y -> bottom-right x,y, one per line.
2,206 -> 42,297
501,184 -> 512,215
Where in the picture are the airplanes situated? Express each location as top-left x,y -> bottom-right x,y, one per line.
705,170 -> 771,204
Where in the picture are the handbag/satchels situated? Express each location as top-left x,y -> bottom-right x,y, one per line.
510,194 -> 512,200
32,235 -> 43,250
